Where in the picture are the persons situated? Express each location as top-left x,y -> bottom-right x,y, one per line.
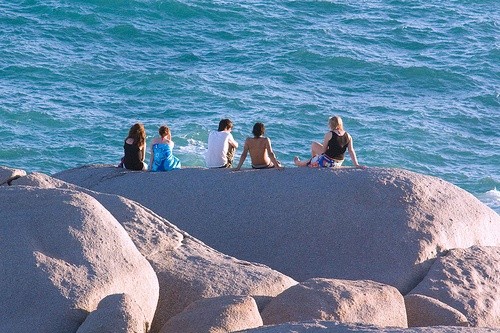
228,122 -> 282,171
118,123 -> 148,172
207,119 -> 238,169
147,125 -> 181,172
294,116 -> 368,169
